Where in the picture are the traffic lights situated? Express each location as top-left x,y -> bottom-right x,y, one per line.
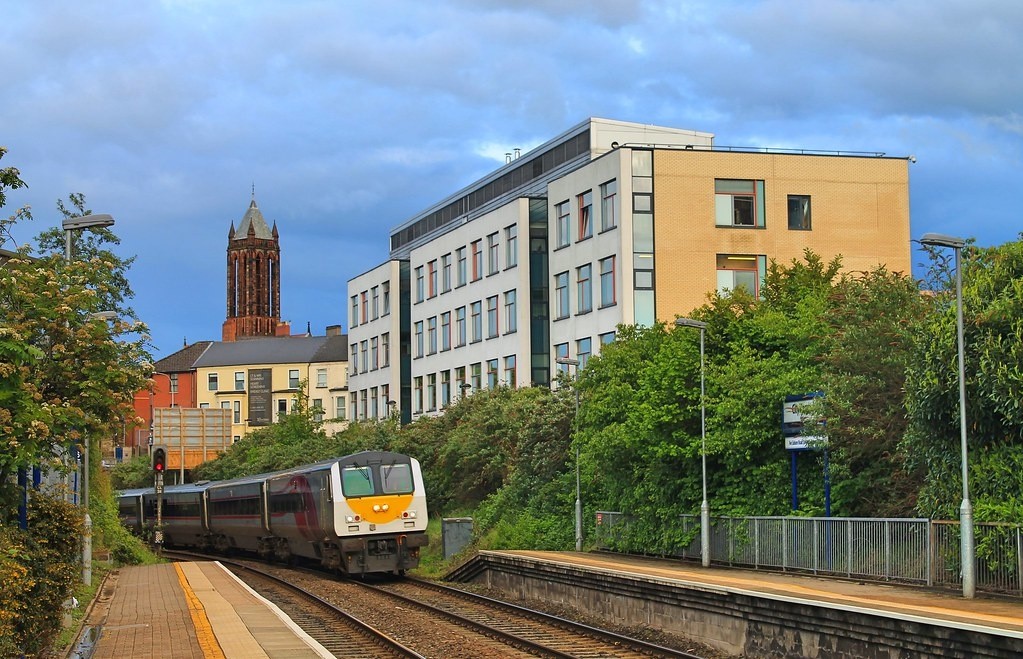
153,447 -> 166,475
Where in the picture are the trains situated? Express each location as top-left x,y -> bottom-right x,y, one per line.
115,449 -> 430,580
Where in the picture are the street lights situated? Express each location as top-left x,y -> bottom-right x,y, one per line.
61,213 -> 115,608
556,357 -> 584,551
919,232 -> 976,602
676,316 -> 712,568
